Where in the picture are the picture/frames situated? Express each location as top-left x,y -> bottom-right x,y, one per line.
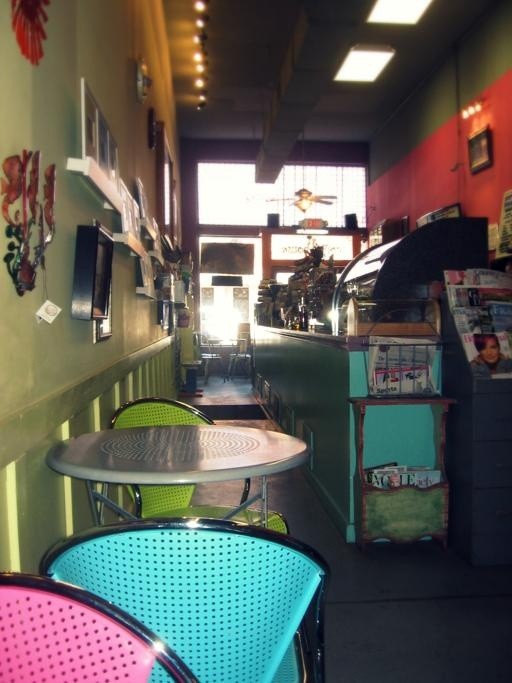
466,127 -> 494,174
65,60 -> 191,346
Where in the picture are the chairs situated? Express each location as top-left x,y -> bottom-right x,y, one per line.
39,519 -> 330,682
0,572 -> 198,682
227,338 -> 252,381
109,397 -> 290,534
200,339 -> 226,384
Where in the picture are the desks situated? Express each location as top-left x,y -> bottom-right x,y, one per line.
46,425 -> 309,529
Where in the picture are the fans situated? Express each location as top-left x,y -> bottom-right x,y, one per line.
265,119 -> 339,214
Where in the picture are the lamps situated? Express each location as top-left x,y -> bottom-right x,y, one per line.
294,190 -> 313,213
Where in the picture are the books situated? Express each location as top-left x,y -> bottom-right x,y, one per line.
444,268 -> 511,381
367,335 -> 434,393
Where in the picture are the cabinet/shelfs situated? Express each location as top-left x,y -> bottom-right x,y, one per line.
348,392 -> 458,552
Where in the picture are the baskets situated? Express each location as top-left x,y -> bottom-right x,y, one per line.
363,308 -> 444,399
